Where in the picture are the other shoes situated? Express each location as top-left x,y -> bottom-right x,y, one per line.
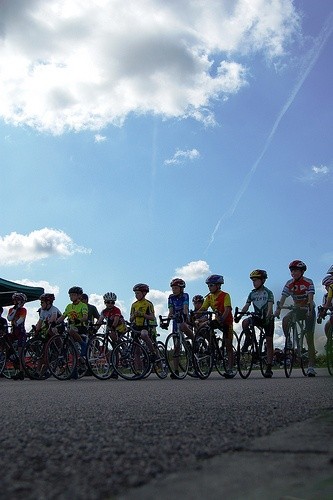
306,368 -> 317,377
10,371 -> 25,381
170,371 -> 179,379
264,370 -> 273,378
226,368 -> 234,376
103,372 -> 118,380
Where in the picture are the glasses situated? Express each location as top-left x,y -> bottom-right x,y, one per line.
290,268 -> 299,272
208,284 -> 216,287
105,301 -> 115,304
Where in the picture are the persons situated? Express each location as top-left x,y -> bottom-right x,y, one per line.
235,269 -> 275,379
161,277 -> 197,378
4,292 -> 28,381
317,264 -> 333,339
51,286 -> 92,376
127,284 -> 167,379
80,294 -> 101,336
34,294 -> 64,376
90,293 -> 126,379
191,275 -> 236,378
188,293 -> 213,355
272,260 -> 317,378
0,307 -> 8,338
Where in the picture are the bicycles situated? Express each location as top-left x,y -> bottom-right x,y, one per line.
316,303 -> 333,376
273,300 -> 319,379
233,305 -> 274,379
0,309 -> 241,379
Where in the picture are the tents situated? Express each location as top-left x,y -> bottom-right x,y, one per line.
0,278 -> 44,308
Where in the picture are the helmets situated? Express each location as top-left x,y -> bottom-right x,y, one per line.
68,287 -> 83,294
288,260 -> 306,271
326,265 -> 333,273
170,278 -> 185,288
250,270 -> 268,279
205,275 -> 224,284
39,293 -> 55,302
133,284 -> 149,292
81,294 -> 88,299
192,295 -> 204,302
12,293 -> 27,302
322,274 -> 333,285
103,292 -> 117,301
